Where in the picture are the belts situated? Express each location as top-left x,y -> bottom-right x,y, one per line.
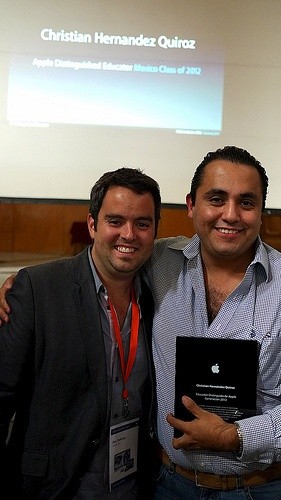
161,449 -> 281,490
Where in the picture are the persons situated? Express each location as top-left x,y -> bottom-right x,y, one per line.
0,167 -> 163,500
0,145 -> 280,500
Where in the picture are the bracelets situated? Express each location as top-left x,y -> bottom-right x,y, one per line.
236,424 -> 245,457
